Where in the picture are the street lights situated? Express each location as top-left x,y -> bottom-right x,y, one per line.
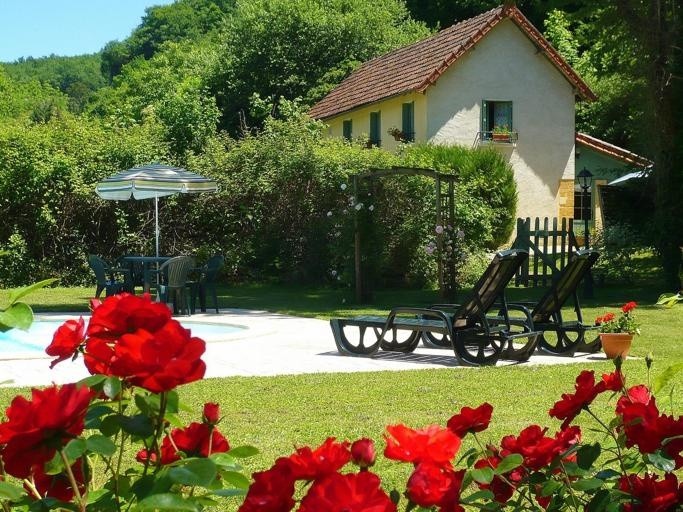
577,165 -> 594,297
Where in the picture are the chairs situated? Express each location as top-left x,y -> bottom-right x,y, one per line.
88,243 -> 224,317
419,250 -> 604,359
330,250 -> 543,367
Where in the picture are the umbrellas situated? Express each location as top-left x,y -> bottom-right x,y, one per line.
94,159 -> 218,303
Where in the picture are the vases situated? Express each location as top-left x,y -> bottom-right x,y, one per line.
493,134 -> 510,142
600,334 -> 633,358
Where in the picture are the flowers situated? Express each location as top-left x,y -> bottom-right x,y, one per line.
492,123 -> 510,134
236,352 -> 683,512
594,300 -> 643,333
0,290 -> 258,512
